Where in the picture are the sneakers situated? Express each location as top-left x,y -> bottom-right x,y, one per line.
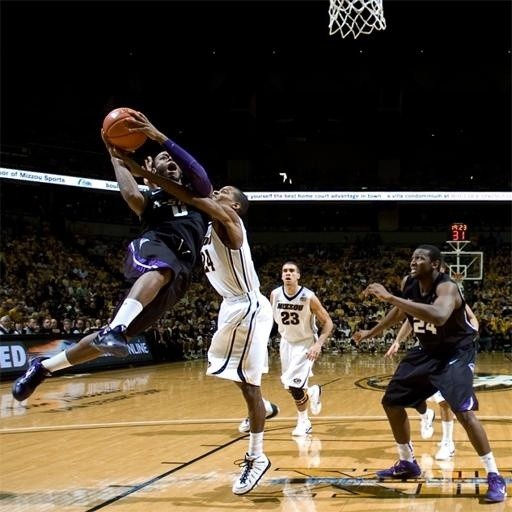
421,409 -> 435,438
309,385 -> 322,415
12,356 -> 50,401
90,325 -> 129,358
232,452 -> 272,496
376,459 -> 421,479
292,421 -> 311,435
239,402 -> 279,435
436,441 -> 455,460
484,472 -> 506,502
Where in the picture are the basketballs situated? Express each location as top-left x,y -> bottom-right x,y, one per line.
102,107 -> 146,151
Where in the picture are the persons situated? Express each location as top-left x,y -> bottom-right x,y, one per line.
144,269 -> 223,359
269,260 -> 334,438
384,261 -> 479,462
352,242 -> 508,503
108,144 -> 279,496
250,240 -> 512,352
0,215 -> 136,337
12,109 -> 214,401
